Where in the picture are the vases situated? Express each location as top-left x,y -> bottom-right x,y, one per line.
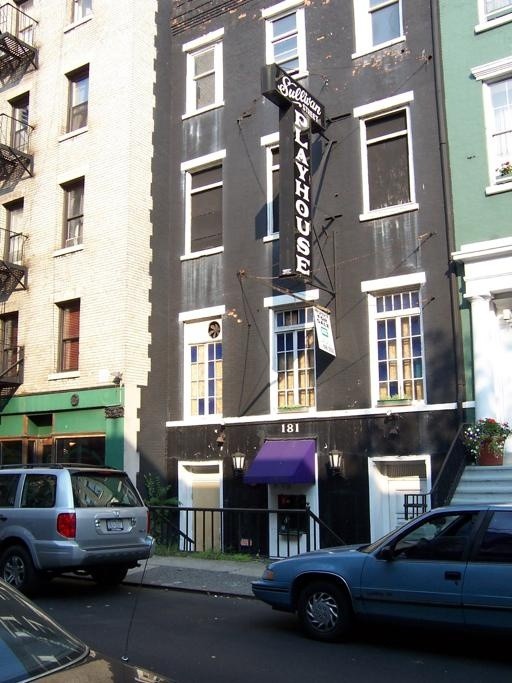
478,439 -> 504,466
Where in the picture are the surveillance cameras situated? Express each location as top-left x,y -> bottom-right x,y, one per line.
503,309 -> 511,323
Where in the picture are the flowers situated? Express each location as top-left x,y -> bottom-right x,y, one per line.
462,418 -> 512,464
496,161 -> 512,182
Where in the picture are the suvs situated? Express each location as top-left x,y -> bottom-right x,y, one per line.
0,461 -> 154,595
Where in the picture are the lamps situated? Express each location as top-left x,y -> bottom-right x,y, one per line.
328,450 -> 346,479
386,409 -> 401,437
231,452 -> 246,478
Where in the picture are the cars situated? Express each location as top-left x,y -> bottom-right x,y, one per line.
250,502 -> 512,654
0,579 -> 178,683
